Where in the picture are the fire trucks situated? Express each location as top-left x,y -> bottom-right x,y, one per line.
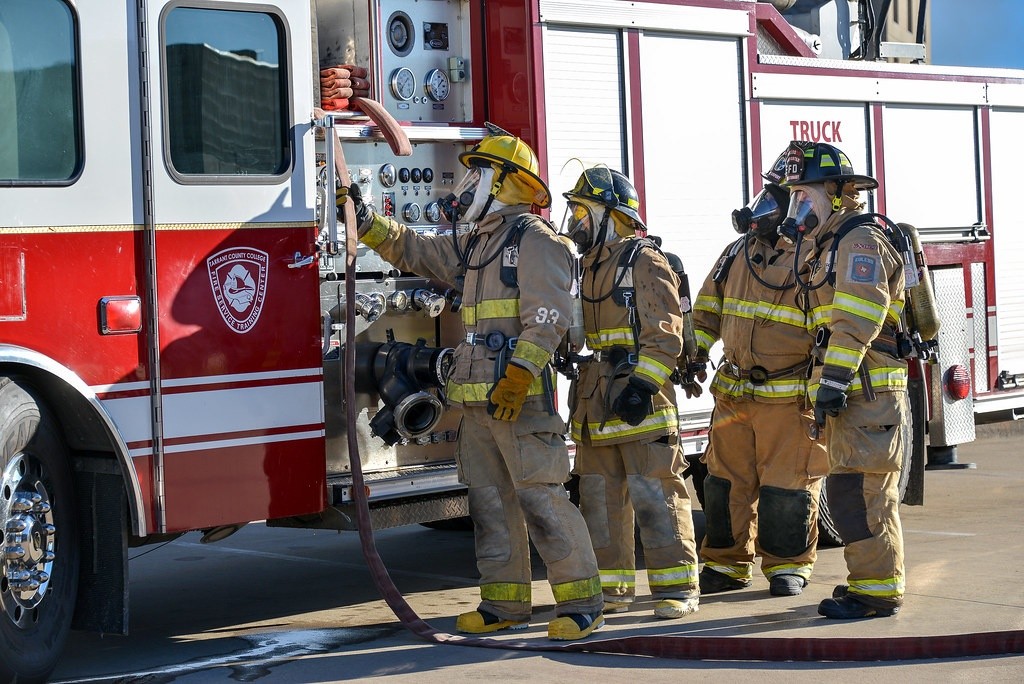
0,0 -> 1024,684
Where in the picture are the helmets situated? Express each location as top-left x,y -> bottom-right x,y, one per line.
759,149 -> 787,186
777,140 -> 879,191
458,135 -> 553,210
563,167 -> 648,232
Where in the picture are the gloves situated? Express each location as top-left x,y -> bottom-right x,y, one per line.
485,362 -> 533,422
612,376 -> 653,426
335,183 -> 373,239
677,354 -> 708,399
813,376 -> 851,428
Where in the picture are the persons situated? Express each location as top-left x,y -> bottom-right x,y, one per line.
777,141 -> 941,618
680,158 -> 827,596
553,167 -> 701,618
339,136 -> 606,641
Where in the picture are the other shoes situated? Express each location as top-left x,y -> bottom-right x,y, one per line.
654,596 -> 700,618
818,596 -> 899,620
698,566 -> 752,594
771,574 -> 806,595
833,584 -> 850,599
455,609 -> 529,633
549,609 -> 606,640
602,600 -> 630,614
